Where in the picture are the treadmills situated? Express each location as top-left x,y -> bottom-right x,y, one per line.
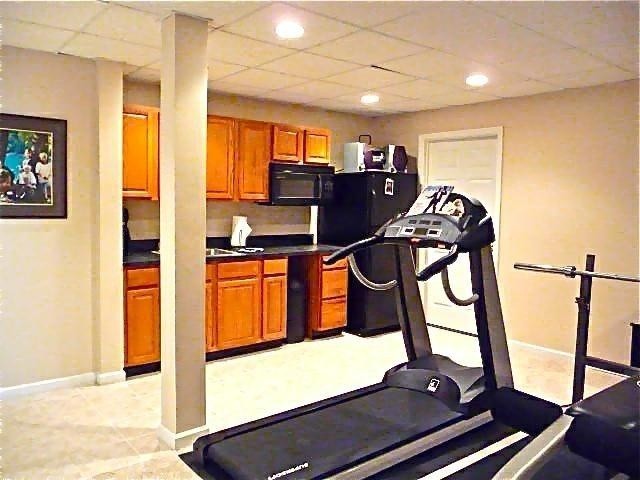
194,188 -> 516,480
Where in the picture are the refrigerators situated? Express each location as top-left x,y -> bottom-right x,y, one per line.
317,170 -> 420,336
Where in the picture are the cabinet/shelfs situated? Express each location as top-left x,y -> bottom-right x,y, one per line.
270,123 -> 331,164
300,254 -> 349,332
206,115 -> 270,202
261,258 -> 288,341
206,261 -> 215,353
123,267 -> 160,367
123,104 -> 159,199
217,260 -> 260,351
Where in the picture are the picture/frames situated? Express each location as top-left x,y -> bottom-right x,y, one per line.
0,113 -> 69,219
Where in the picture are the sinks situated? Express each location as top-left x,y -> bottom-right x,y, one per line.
151,247 -> 247,257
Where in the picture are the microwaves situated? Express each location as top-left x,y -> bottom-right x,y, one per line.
257,162 -> 336,207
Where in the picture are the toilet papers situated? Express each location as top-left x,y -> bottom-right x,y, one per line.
230,215 -> 253,247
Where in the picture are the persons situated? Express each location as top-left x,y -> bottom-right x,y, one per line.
0,149 -> 51,203
